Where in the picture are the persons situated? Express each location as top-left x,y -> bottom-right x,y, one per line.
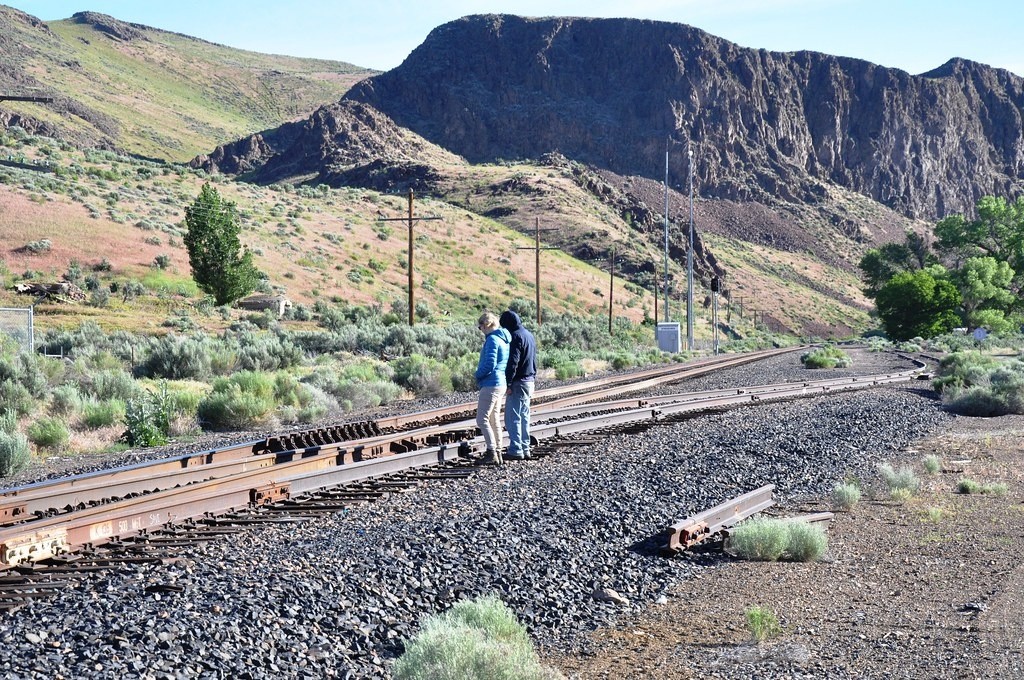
474,314 -> 511,465
499,311 -> 537,459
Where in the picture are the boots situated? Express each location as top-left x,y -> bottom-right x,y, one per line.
497,451 -> 503,464
475,448 -> 499,464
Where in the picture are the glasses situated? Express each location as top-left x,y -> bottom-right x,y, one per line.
478,324 -> 484,330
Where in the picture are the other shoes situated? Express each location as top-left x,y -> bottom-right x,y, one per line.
503,453 -> 524,460
525,455 -> 532,460
